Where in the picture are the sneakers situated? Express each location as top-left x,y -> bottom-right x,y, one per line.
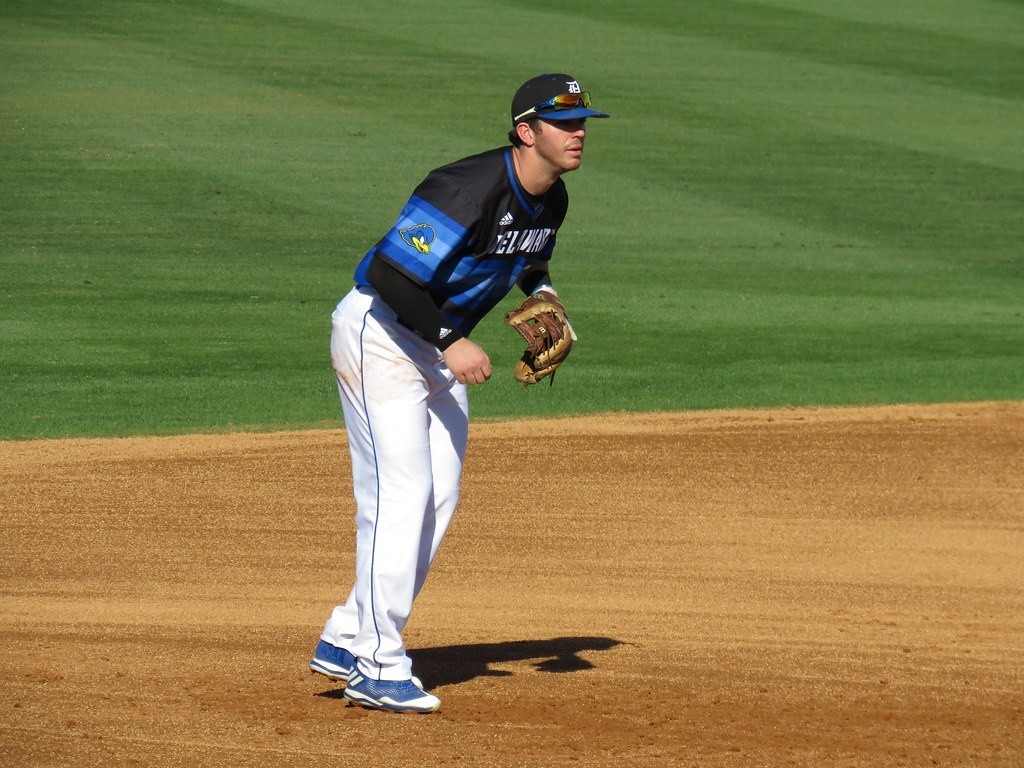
342,659 -> 442,713
309,640 -> 423,691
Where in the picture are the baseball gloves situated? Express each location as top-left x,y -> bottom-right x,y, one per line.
504,291 -> 578,387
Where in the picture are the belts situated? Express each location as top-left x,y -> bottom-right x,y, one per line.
395,315 -> 430,340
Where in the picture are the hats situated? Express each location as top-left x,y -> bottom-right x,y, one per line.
511,74 -> 611,127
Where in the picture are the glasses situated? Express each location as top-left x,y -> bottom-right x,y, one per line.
514,91 -> 591,122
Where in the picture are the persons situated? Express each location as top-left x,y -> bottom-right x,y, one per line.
309,76 -> 613,716
